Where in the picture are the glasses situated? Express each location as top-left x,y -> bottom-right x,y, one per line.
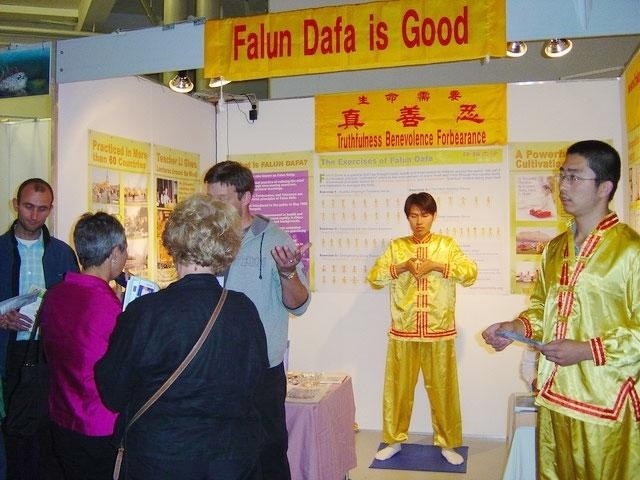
555,173 -> 602,183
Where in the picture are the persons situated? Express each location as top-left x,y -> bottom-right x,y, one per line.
93,193 -> 271,479
161,186 -> 170,207
1,177 -> 80,480
39,212 -> 128,480
481,139 -> 640,479
203,159 -> 312,480
365,191 -> 477,466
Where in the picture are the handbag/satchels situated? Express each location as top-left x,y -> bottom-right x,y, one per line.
98,413 -> 129,480
0,359 -> 49,438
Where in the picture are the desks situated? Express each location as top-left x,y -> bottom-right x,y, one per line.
285,372 -> 357,480
502,391 -> 540,480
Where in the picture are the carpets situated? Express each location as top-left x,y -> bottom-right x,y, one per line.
369,442 -> 469,473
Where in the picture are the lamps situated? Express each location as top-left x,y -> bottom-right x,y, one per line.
168,71 -> 195,93
507,42 -> 528,58
208,77 -> 231,104
544,38 -> 573,58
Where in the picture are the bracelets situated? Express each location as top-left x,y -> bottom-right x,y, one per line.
278,270 -> 296,280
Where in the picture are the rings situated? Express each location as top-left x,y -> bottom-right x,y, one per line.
289,260 -> 294,264
